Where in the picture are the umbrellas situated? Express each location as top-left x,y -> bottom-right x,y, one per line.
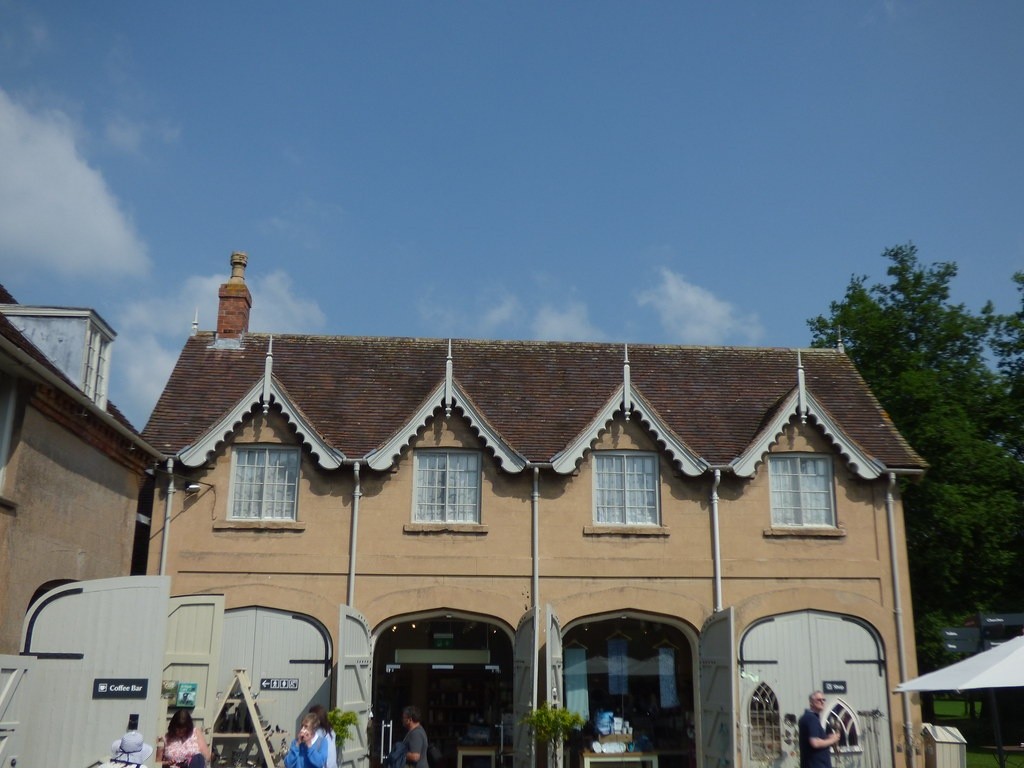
892,635 -> 1024,694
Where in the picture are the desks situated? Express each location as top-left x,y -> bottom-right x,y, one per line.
645,750 -> 688,768
456,744 -> 498,768
578,750 -> 661,768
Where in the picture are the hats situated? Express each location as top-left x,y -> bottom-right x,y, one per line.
110,732 -> 154,763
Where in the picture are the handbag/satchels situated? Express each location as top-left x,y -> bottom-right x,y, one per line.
427,744 -> 443,763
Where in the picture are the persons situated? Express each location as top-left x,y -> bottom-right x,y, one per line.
156,710 -> 208,768
393,705 -> 429,768
284,705 -> 337,768
798,691 -> 840,768
97,732 -> 153,768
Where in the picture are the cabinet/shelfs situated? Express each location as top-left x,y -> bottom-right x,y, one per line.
428,704 -> 483,740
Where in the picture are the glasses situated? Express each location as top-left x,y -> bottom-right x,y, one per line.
815,699 -> 826,702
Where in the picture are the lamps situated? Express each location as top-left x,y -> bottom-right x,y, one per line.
185,482 -> 201,493
